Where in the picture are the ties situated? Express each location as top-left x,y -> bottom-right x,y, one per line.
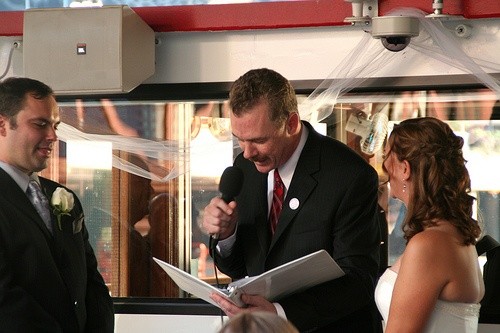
28,180 -> 53,237
268,168 -> 285,240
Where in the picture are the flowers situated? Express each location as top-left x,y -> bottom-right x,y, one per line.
51,187 -> 74,231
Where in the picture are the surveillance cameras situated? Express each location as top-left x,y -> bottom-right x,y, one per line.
372,16 -> 420,52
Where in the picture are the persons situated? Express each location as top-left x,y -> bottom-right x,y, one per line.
127,144 -> 183,299
373,117 -> 485,333
219,311 -> 299,333
203,68 -> 385,333
0,77 -> 116,333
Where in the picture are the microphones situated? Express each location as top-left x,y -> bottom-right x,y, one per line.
210,167 -> 243,249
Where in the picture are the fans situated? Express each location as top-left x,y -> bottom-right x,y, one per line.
344,103 -> 388,155
